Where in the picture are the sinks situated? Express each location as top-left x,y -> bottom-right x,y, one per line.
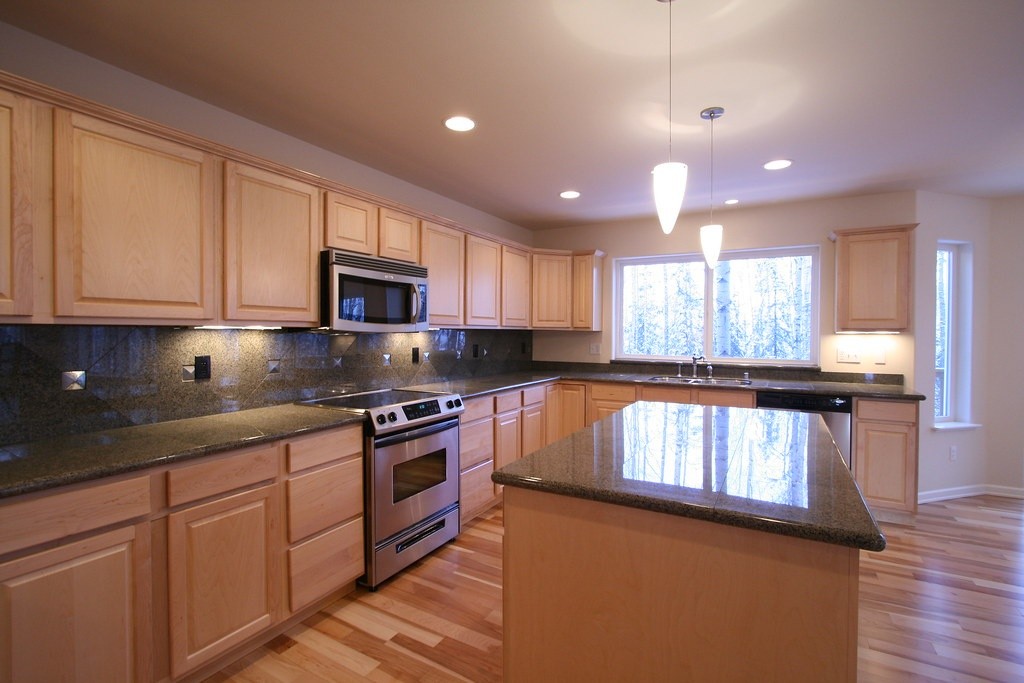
648,376 -> 691,384
691,379 -> 753,386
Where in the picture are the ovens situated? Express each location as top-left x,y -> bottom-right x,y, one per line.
363,419 -> 459,592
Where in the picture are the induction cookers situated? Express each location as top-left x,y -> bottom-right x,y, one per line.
294,384 -> 465,436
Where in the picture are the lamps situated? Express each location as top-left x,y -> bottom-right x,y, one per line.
700,107 -> 724,270
652,0 -> 688,234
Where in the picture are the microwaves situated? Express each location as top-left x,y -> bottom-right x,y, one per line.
280,249 -> 429,336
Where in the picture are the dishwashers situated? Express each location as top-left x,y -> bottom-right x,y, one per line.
755,391 -> 851,470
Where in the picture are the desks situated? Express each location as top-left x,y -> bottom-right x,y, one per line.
491,400 -> 887,683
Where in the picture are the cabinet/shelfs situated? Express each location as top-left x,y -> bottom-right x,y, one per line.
0,71 -> 606,331
851,397 -> 919,526
0,420 -> 366,683
459,380 -> 757,526
831,223 -> 920,330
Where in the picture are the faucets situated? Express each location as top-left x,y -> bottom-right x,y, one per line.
692,356 -> 705,378
